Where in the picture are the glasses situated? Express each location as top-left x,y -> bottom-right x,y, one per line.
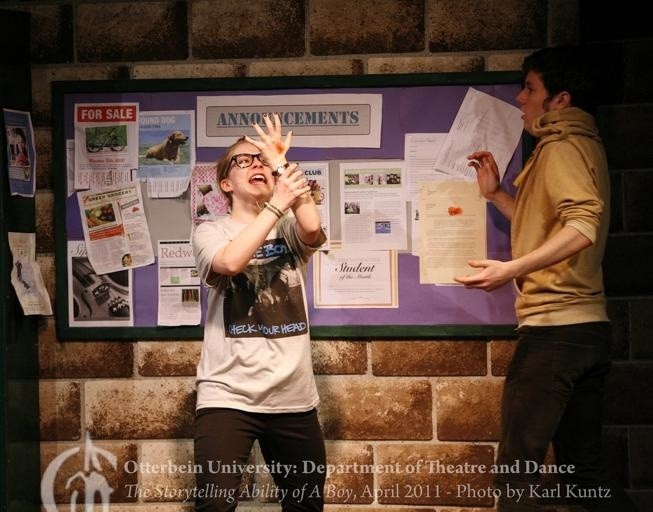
225,153 -> 269,179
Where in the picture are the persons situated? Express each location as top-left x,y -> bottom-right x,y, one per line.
343,171 -> 400,185
308,179 -> 323,205
449,44 -> 615,511
190,110 -> 328,512
232,259 -> 292,319
11,127 -> 30,167
344,202 -> 360,214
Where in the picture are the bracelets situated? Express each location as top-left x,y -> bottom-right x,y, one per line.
263,201 -> 284,219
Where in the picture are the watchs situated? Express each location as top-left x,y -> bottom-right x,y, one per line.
271,162 -> 290,177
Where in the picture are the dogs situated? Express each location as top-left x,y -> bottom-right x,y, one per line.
146,130 -> 189,165
